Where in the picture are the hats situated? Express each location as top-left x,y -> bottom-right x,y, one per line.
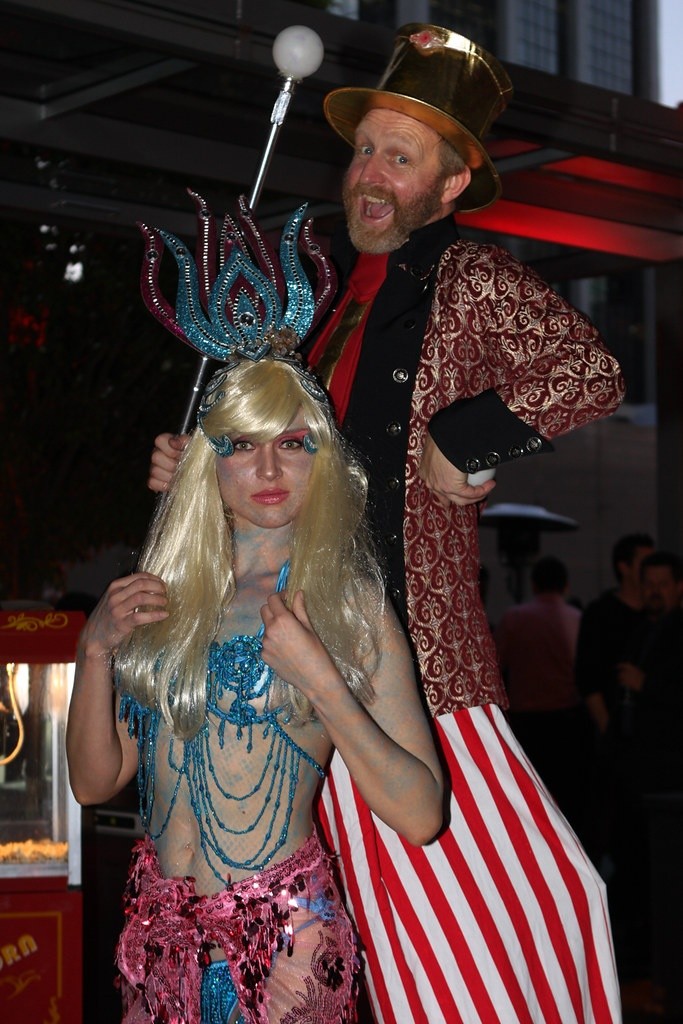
323,20 -> 513,214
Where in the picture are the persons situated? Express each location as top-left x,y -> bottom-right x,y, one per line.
482,531 -> 683,984
64,358 -> 448,1023
150,23 -> 624,1023
